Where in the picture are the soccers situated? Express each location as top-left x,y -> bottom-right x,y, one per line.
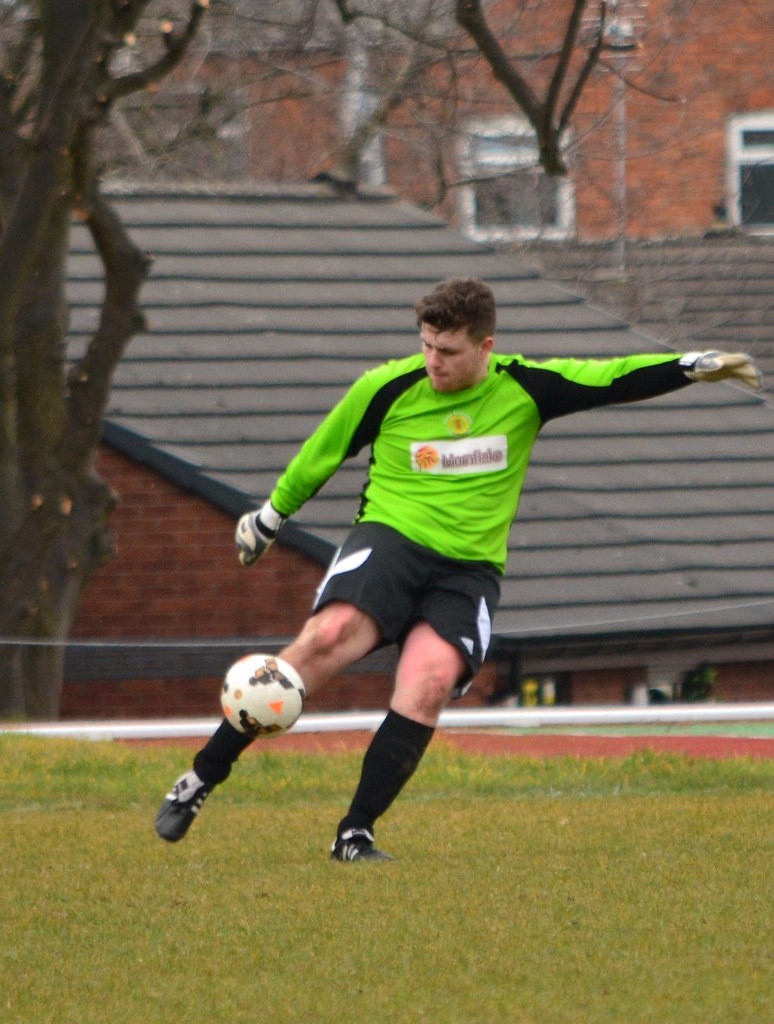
220,654 -> 307,740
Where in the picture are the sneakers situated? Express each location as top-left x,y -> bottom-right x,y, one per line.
331,827 -> 390,862
155,770 -> 214,842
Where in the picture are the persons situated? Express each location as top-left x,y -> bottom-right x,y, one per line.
154,275 -> 765,864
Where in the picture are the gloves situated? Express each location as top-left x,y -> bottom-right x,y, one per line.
235,499 -> 289,569
678,349 -> 762,389
